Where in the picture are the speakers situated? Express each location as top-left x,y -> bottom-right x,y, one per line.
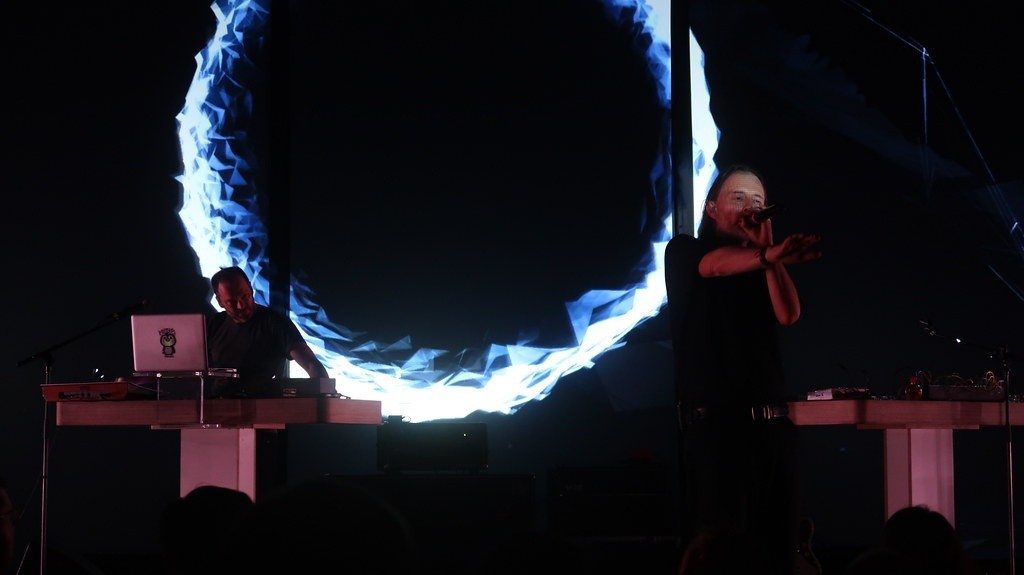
323,471 -> 538,554
542,464 -> 677,541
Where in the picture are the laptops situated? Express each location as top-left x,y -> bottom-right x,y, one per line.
130,314 -> 237,373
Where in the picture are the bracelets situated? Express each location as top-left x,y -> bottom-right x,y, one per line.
760,244 -> 774,270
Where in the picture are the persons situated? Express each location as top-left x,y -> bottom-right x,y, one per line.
666,165 -> 822,575
0,475 -> 19,575
129,266 -> 338,510
846,507 -> 966,575
160,483 -> 421,575
681,518 -> 821,575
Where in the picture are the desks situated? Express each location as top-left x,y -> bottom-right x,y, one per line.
787,400 -> 1024,575
55,398 -> 381,575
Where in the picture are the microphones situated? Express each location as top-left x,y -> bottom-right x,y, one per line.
746,204 -> 782,228
109,299 -> 148,320
